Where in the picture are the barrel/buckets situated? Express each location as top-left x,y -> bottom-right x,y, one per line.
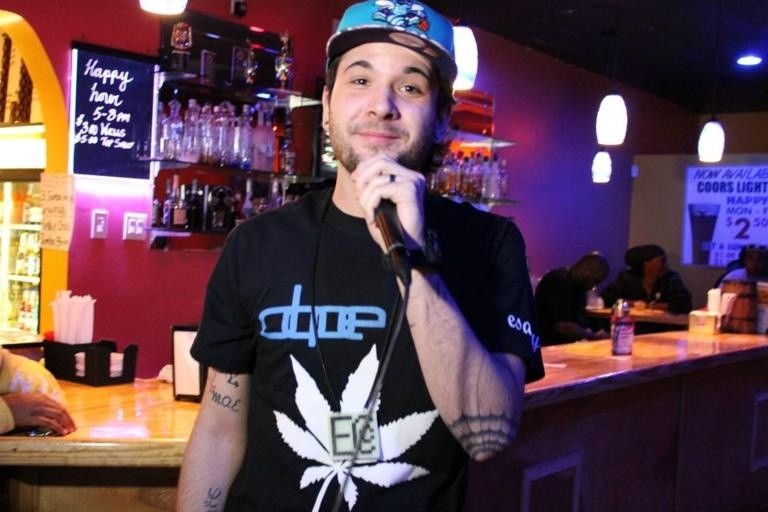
721,280 -> 756,332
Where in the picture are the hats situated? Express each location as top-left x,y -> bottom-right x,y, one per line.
326,0 -> 457,83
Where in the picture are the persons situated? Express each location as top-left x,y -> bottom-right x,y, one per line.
713,244 -> 756,288
601,245 -> 692,314
533,252 -> 610,344
0,344 -> 76,438
168,0 -> 546,512
716,245 -> 768,290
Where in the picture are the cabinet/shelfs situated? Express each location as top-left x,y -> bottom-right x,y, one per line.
142,9 -> 327,253
310,76 -> 520,207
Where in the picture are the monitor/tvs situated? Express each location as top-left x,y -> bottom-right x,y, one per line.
312,75 -> 337,179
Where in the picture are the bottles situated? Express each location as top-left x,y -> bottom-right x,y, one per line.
427,148 -> 504,200
152,7 -> 294,238
6,187 -> 42,334
610,298 -> 634,356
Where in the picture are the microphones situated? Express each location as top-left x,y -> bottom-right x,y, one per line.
374,200 -> 414,285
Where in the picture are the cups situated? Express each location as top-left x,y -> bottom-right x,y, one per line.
688,203 -> 721,265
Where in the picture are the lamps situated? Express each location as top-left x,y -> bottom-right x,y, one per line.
448,1 -> 479,92
698,0 -> 726,165
139,0 -> 188,17
595,0 -> 629,146
591,47 -> 613,184
